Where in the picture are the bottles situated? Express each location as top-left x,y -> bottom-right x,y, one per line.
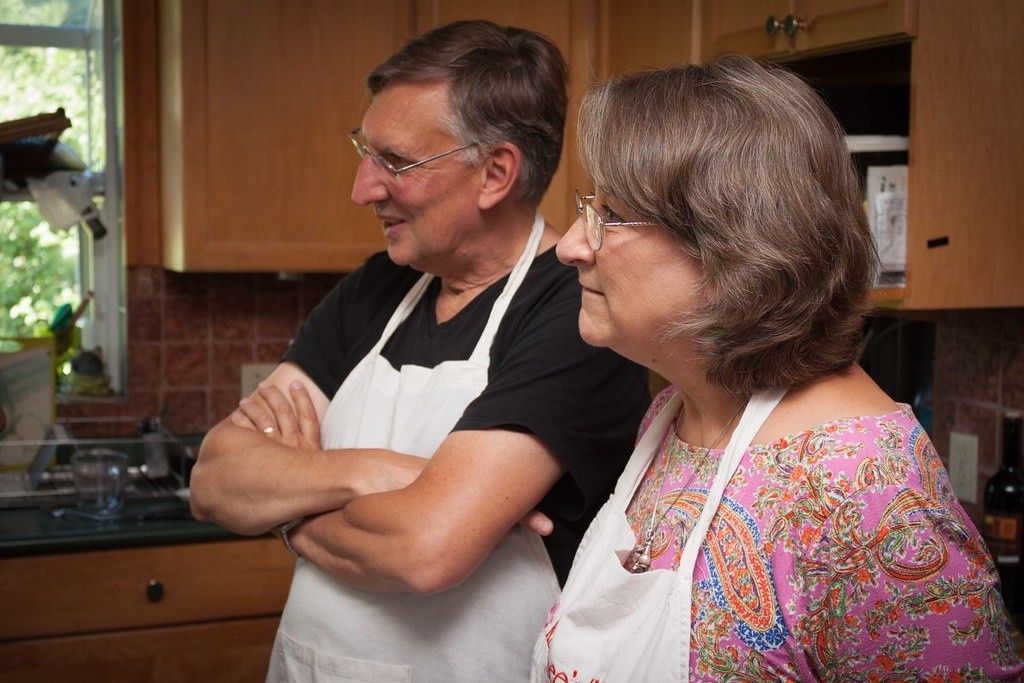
979,410 -> 1024,625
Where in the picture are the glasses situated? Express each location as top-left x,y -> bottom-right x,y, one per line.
574,188 -> 662,251
347,126 -> 485,186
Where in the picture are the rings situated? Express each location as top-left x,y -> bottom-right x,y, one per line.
263,426 -> 278,435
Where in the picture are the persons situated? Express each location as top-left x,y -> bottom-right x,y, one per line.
529,52 -> 1024,683
188,19 -> 652,683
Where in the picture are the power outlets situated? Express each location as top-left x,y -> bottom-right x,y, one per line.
948,433 -> 980,504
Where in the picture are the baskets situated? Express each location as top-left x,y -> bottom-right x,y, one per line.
54,398 -> 147,439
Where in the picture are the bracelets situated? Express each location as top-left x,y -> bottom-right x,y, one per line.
281,519 -> 302,559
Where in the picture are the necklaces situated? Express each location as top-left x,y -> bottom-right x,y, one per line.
631,390 -> 751,573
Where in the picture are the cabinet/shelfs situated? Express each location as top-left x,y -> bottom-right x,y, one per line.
122,0 -> 1024,313
0,539 -> 297,683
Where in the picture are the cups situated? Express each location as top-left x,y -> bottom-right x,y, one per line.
71,450 -> 127,516
